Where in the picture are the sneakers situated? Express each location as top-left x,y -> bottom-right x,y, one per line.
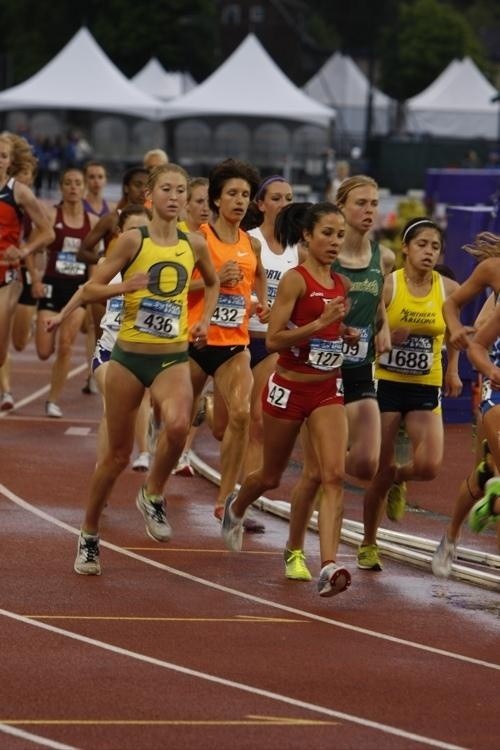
386,463 -> 407,523
136,484 -> 172,543
356,544 -> 384,570
469,439 -> 499,534
282,547 -> 352,598
0,372 -> 99,419
73,527 -> 101,576
133,394 -> 206,477
432,524 -> 461,577
213,492 -> 265,553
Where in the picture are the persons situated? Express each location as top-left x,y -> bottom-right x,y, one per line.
2,130 -> 500,598
